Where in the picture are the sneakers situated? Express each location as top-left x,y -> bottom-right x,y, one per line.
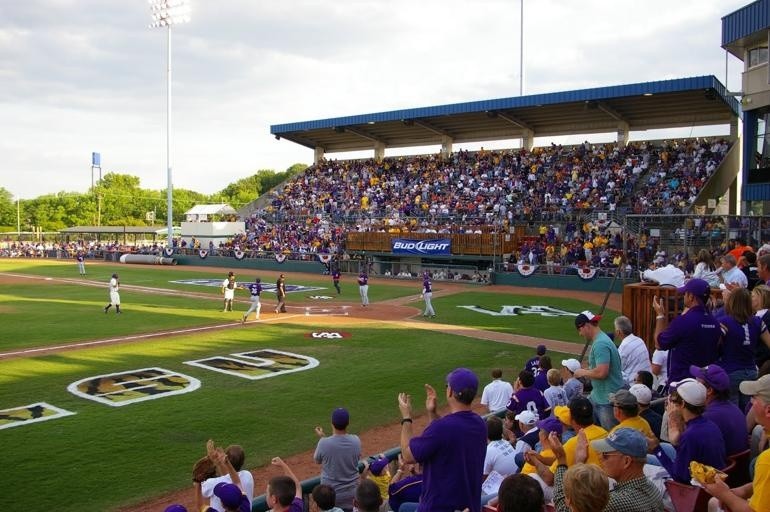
274,309 -> 287,314
221,306 -> 234,313
241,314 -> 260,325
422,314 -> 438,319
103,306 -> 122,315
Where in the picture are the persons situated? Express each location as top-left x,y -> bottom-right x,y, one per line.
220,138 -> 733,273
262,455 -> 304,512
201,438 -> 255,511
273,272 -> 290,316
1,236 -> 215,263
194,444 -> 253,511
104,273 -> 123,314
76,247 -> 87,278
241,277 -> 265,324
307,234 -> 769,512
221,270 -> 238,315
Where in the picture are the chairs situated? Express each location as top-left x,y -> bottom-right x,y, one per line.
719,461 -> 738,489
728,447 -> 752,486
663,478 -> 711,512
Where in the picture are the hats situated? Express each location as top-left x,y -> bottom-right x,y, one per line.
447,367 -> 480,390
739,373 -> 770,402
688,362 -> 731,389
331,407 -> 349,424
677,278 -> 712,296
370,457 -> 389,474
589,427 -> 648,458
561,357 -> 581,373
608,383 -> 653,406
668,377 -> 708,408
574,310 -> 602,327
213,482 -> 241,503
512,410 -> 537,424
536,417 -> 565,435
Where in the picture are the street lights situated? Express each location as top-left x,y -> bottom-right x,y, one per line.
145,0 -> 193,246
